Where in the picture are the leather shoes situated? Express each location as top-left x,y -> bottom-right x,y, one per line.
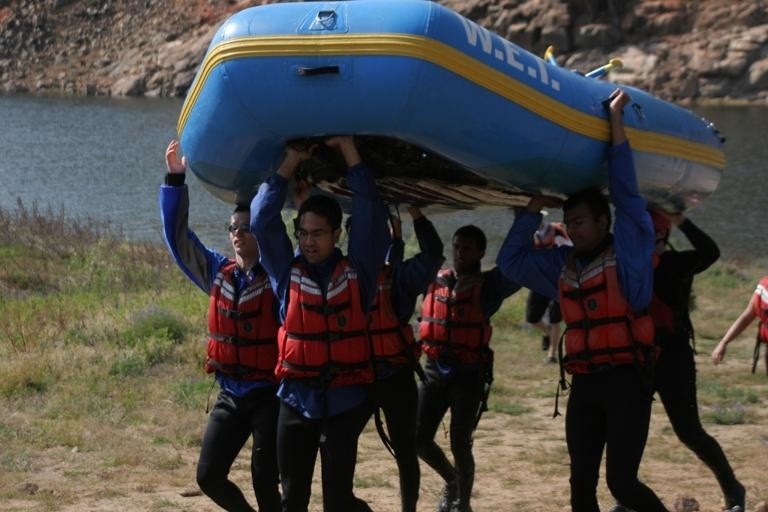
543,325 -> 551,350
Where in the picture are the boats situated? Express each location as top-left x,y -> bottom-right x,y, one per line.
172,1 -> 728,223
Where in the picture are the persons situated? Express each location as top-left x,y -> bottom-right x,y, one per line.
708,275 -> 767,368
629,198 -> 747,512
247,134 -> 389,511
493,87 -> 668,511
160,138 -> 283,512
403,192 -> 526,512
525,203 -> 572,363
293,180 -> 446,510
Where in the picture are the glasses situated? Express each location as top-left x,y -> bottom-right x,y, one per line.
228,223 -> 250,233
294,229 -> 328,243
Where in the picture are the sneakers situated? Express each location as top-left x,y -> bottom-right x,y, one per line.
438,480 -> 457,512
454,501 -> 472,512
724,485 -> 746,512
